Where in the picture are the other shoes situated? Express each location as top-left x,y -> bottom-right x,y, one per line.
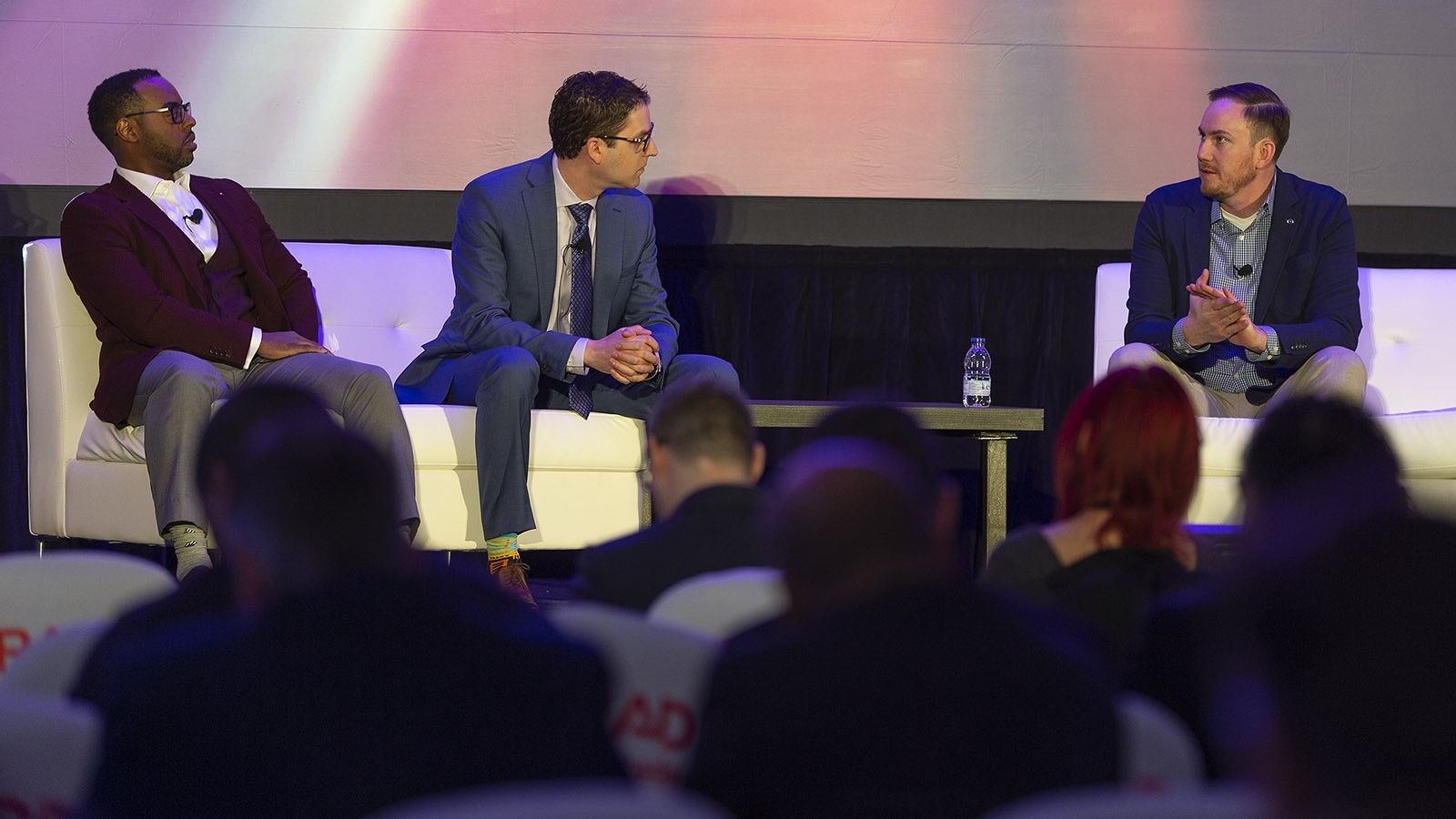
176,565 -> 211,587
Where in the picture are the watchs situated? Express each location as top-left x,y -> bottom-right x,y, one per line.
645,351 -> 661,380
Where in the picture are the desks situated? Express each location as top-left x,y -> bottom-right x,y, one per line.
744,404 -> 1044,585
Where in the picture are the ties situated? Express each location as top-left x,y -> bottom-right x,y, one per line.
567,203 -> 593,420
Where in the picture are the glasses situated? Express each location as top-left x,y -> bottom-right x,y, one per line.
637,438 -> 667,491
115,102 -> 191,137
581,122 -> 654,152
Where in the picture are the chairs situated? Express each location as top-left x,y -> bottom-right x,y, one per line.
0,552 -> 1281,819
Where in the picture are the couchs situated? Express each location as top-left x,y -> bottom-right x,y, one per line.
22,237 -> 652,550
1092,263 -> 1456,525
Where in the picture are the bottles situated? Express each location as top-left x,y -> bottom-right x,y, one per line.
961,338 -> 991,408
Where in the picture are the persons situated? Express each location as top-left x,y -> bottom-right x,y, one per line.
59,67 -> 419,583
62,364 -> 1456,819
1107,82 -> 1367,414
394,72 -> 739,614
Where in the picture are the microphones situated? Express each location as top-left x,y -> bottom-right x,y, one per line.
188,208 -> 205,225
574,237 -> 591,254
1237,262 -> 1255,279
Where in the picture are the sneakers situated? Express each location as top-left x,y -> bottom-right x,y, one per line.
484,563 -> 539,610
